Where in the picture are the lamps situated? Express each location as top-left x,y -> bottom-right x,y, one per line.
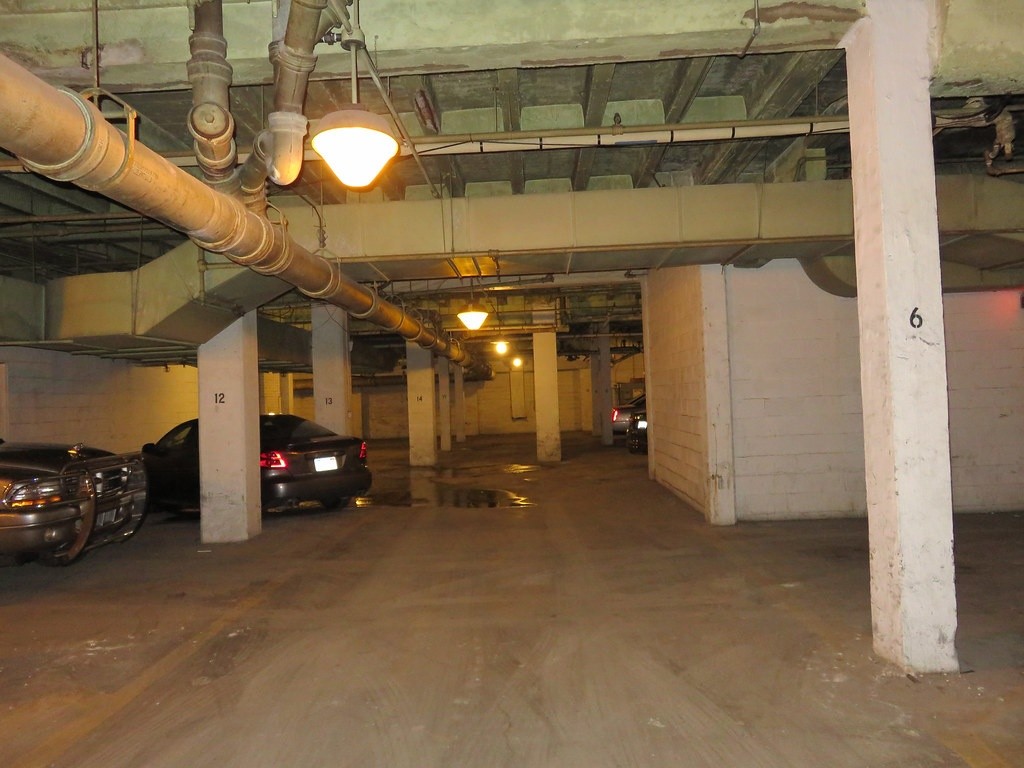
456,277 -> 489,329
310,43 -> 399,186
491,334 -> 509,354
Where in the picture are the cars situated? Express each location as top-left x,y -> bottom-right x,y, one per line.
0,437 -> 150,569
139,412 -> 374,516
611,392 -> 648,456
